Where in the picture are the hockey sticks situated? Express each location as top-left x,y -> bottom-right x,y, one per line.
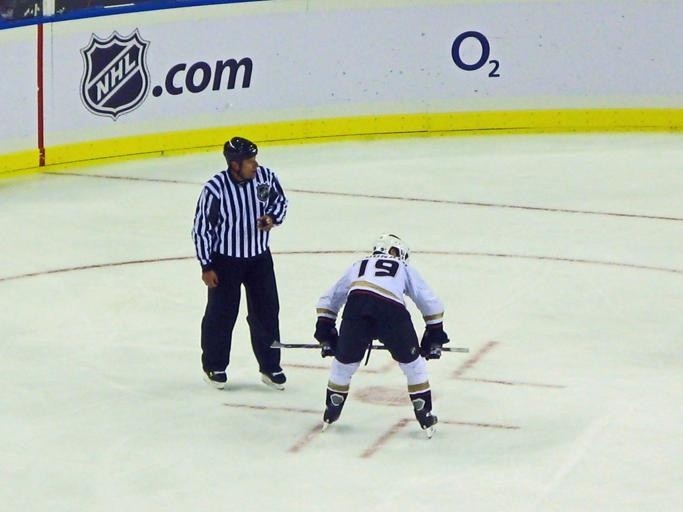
246,315 -> 469,353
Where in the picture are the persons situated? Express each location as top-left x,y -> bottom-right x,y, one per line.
190,137 -> 288,386
313,232 -> 450,429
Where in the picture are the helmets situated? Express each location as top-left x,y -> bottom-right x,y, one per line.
372,232 -> 410,262
223,136 -> 258,162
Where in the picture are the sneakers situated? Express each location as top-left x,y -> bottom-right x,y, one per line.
259,368 -> 286,384
324,410 -> 334,424
203,366 -> 227,382
420,415 -> 438,430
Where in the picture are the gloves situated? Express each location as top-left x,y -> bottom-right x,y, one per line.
420,321 -> 450,360
314,316 -> 338,358
